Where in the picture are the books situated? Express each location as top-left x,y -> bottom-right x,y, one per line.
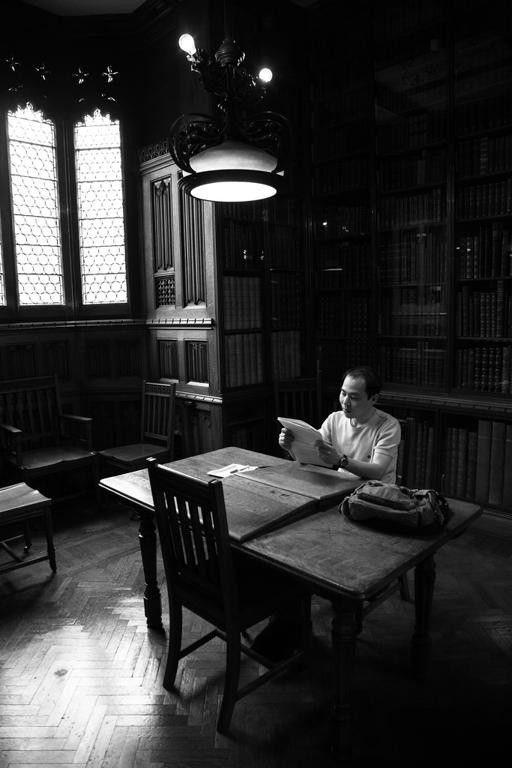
216,31 -> 511,514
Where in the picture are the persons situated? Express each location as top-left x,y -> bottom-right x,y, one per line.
252,360 -> 405,663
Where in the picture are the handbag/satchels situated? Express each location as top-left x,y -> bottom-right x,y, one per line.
338,479 -> 454,538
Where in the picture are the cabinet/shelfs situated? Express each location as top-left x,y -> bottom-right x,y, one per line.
304,1 -> 512,514
137,134 -> 306,455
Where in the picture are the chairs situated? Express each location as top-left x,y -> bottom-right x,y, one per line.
103,379 -> 180,522
0,372 -> 101,501
0,479 -> 58,581
144,452 -> 314,740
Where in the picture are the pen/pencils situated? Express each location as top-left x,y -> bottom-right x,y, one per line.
231,465 -> 249,473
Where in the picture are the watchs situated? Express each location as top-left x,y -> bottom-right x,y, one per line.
339,453 -> 349,468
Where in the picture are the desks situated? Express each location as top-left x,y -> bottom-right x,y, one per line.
96,445 -> 483,767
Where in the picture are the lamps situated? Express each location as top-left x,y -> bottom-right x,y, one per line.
167,25 -> 293,205
